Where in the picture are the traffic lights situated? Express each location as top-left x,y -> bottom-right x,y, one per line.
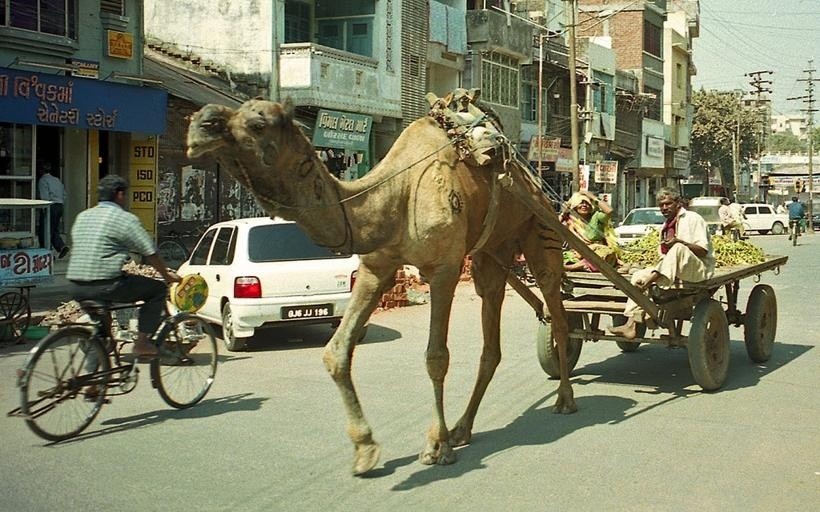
794,180 -> 801,193
800,181 -> 806,193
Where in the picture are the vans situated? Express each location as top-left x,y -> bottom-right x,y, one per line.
688,196 -> 732,237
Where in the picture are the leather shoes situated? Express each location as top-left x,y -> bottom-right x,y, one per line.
58,247 -> 69,258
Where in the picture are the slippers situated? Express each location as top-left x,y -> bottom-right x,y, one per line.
138,343 -> 172,360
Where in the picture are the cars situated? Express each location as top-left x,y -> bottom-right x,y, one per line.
806,212 -> 820,231
168,213 -> 371,353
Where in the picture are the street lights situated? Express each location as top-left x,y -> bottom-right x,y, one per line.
560,9 -> 614,197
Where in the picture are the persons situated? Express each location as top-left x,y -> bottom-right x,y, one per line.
605,186 -> 716,340
718,197 -> 749,240
65,172 -> 182,404
37,156 -> 69,260
777,202 -> 787,214
558,188 -> 618,272
787,196 -> 804,240
727,196 -> 748,221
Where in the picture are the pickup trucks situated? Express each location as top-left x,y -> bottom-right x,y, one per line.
741,203 -> 790,235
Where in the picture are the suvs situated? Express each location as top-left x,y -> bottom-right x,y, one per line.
614,205 -> 667,246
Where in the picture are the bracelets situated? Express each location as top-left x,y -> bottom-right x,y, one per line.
560,211 -> 565,217
595,197 -> 601,205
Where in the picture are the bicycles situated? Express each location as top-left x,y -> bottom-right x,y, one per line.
729,222 -> 752,244
4,262 -> 219,442
155,218 -> 237,269
0,283 -> 35,346
791,219 -> 800,246
